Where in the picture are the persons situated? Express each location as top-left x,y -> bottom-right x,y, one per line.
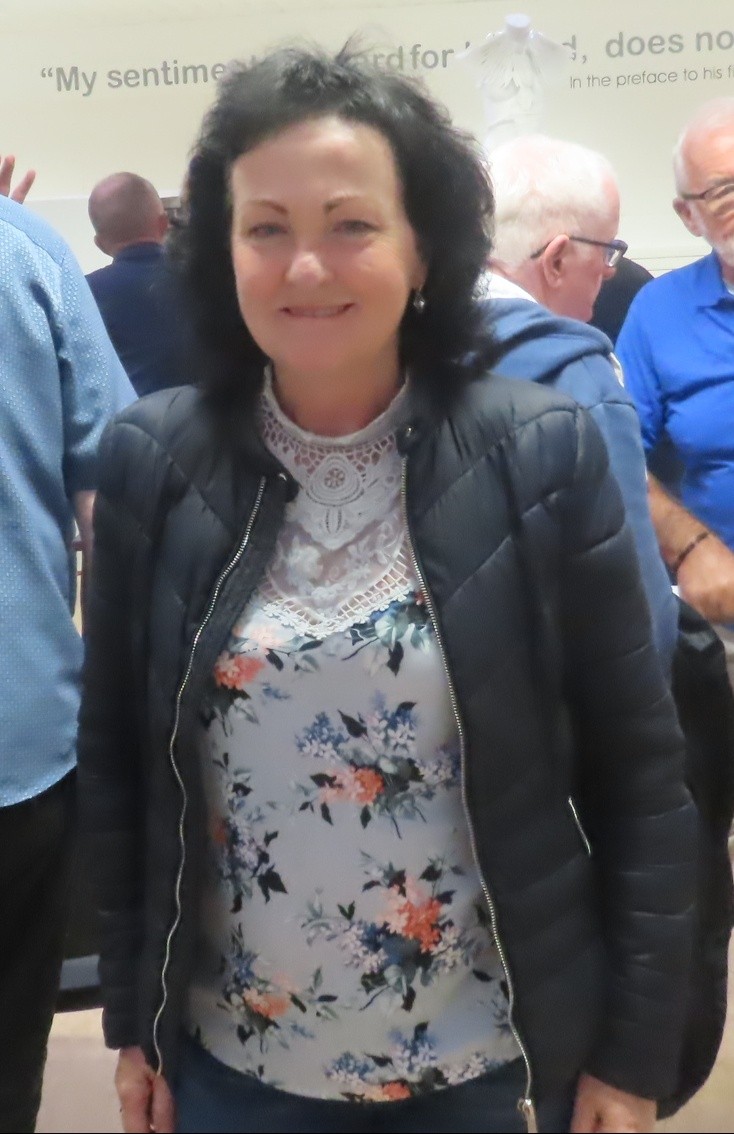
0,53 -> 734,1133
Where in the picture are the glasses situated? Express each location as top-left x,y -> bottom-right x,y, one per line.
531,236 -> 629,268
681,181 -> 734,222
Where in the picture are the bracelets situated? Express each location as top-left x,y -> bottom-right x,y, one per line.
671,531 -> 710,575
